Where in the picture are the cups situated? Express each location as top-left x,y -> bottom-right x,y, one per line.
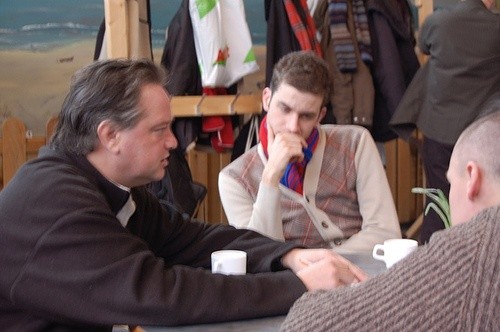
210,249 -> 247,275
373,238 -> 418,269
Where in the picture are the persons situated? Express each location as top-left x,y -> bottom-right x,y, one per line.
218,51 -> 403,254
281,109 -> 500,331
390,0 -> 500,244
0,57 -> 370,332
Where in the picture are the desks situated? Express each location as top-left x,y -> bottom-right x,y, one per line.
132,314 -> 287,331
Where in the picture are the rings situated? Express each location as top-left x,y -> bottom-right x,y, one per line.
349,274 -> 361,289
